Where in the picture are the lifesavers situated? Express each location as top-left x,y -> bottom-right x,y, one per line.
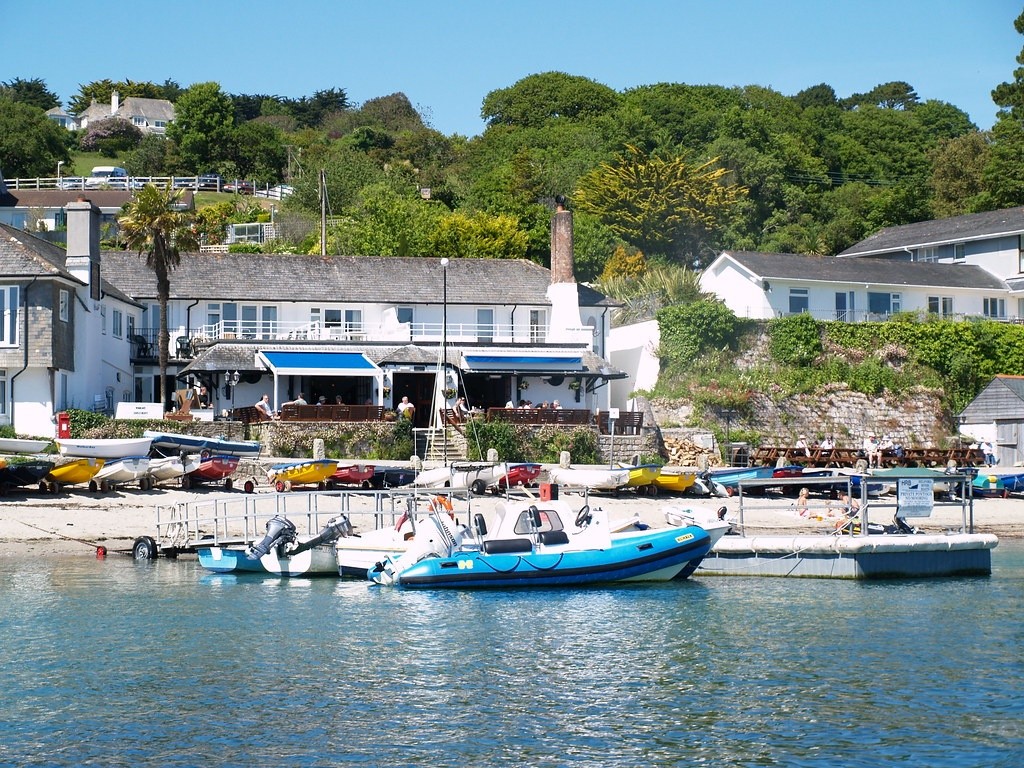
428,496 -> 454,521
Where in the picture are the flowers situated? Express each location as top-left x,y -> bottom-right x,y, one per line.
568,380 -> 580,389
442,388 -> 457,399
518,380 -> 529,391
383,386 -> 390,400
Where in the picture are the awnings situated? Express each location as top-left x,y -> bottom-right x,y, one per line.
255,351 -> 385,375
461,356 -> 583,372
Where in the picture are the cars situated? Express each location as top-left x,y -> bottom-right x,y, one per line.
192,174 -> 224,190
224,180 -> 256,194
173,177 -> 195,191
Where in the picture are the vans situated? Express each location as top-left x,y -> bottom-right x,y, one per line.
84,166 -> 127,191
55,176 -> 88,190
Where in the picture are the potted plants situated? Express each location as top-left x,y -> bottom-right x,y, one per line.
164,409 -> 194,421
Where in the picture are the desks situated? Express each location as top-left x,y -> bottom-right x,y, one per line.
345,331 -> 373,342
190,409 -> 214,422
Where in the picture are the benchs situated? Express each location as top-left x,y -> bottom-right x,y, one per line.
233,406 -> 272,423
281,403 -> 385,422
438,408 -> 467,424
486,407 -> 590,423
596,410 -> 644,436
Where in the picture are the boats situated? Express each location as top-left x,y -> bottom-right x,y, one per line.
0,438 -> 1024,597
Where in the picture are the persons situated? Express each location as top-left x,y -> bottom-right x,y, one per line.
255,394 -> 278,421
282,393 -> 307,416
795,488 -> 817,519
452,397 -> 471,422
314,396 -> 348,421
504,398 -> 564,422
197,387 -> 208,409
748,433 -> 996,474
395,397 -> 414,421
839,491 -> 859,519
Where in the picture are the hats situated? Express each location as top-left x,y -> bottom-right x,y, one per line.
319,396 -> 326,401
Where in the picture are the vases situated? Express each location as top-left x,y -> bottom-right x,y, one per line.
522,386 -> 528,389
376,389 -> 391,398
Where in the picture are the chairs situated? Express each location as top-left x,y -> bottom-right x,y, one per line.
132,334 -> 154,358
175,336 -> 191,359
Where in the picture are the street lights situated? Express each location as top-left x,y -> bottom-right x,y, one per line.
58,160 -> 65,178
440,257 -> 452,467
